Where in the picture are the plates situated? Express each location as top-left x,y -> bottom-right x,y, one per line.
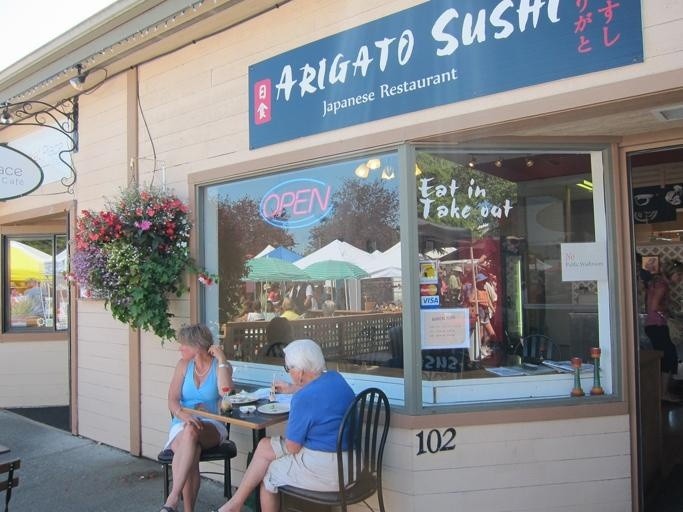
258,403 -> 292,415
227,391 -> 260,404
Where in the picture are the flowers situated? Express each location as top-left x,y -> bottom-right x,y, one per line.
71,181 -> 216,349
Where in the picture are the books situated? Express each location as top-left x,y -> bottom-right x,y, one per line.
541,360 -> 594,373
545,364 -> 573,374
484,363 -> 554,377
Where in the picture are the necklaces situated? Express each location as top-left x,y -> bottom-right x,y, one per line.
194,357 -> 212,377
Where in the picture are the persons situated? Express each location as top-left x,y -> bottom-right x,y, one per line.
634,253 -> 683,407
213,338 -> 362,512
8,278 -> 49,326
237,300 -> 303,322
159,323 -> 232,511
436,254 -> 495,358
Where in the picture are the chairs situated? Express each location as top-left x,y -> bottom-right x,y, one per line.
158,404 -> 239,505
278,387 -> 391,512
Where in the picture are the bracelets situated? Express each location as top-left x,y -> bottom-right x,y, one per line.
173,409 -> 181,416
216,364 -> 230,368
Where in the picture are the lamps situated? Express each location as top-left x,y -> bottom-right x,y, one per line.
1,102 -> 14,125
70,64 -> 90,95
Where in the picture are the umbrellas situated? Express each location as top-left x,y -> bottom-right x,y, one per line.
418,245 -> 497,268
240,238 -> 425,317
42,249 -> 67,279
7,240 -> 53,283
